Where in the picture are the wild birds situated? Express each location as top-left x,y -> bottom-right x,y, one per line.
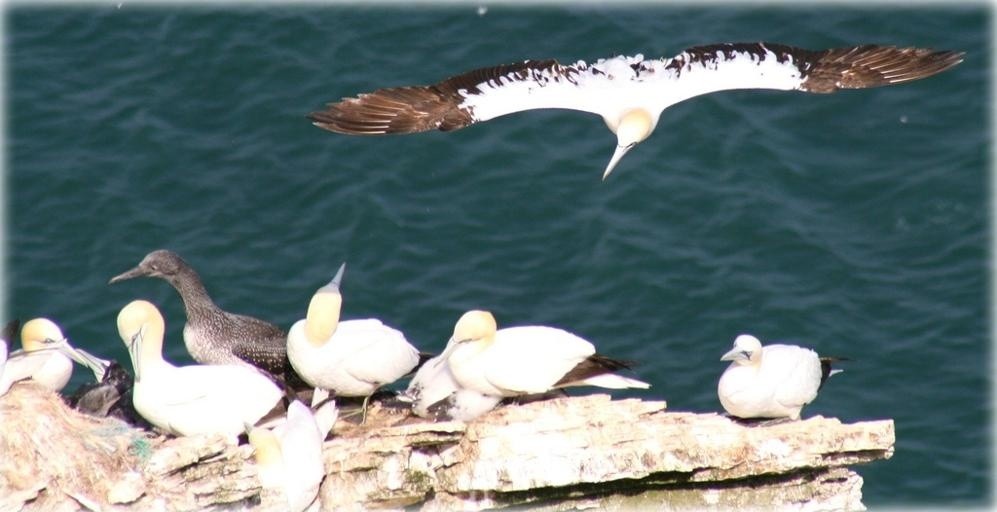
108,246 -> 297,408
305,42 -> 970,182
286,262 -> 435,426
62,344 -> 158,434
398,344 -> 510,421
715,333 -> 849,425
0,316 -> 93,401
112,298 -> 286,453
433,311 -> 655,405
